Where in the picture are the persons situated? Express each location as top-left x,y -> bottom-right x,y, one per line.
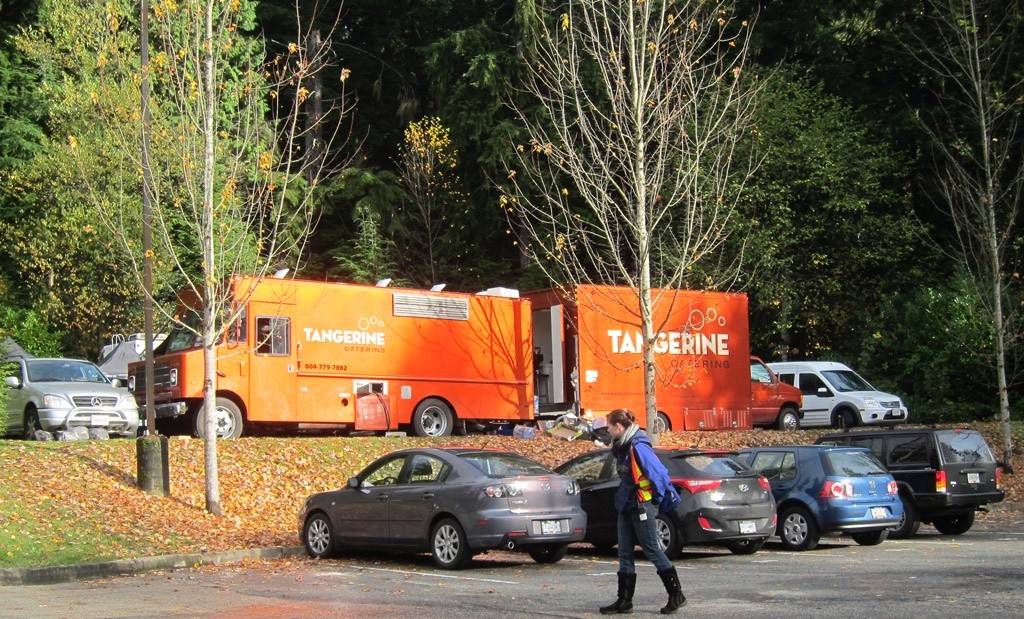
600,410 -> 687,614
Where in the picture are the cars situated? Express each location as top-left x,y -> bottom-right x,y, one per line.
297,445 -> 589,572
1,355 -> 140,440
700,444 -> 904,554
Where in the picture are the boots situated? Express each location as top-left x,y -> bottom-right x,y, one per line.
599,572 -> 637,614
656,564 -> 687,614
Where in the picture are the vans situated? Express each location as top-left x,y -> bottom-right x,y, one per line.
96,331 -> 169,392
750,360 -> 908,429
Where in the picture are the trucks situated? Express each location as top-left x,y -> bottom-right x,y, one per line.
125,273 -> 536,441
522,282 -> 805,436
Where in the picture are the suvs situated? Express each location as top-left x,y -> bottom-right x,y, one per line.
550,447 -> 778,560
811,425 -> 1005,540
